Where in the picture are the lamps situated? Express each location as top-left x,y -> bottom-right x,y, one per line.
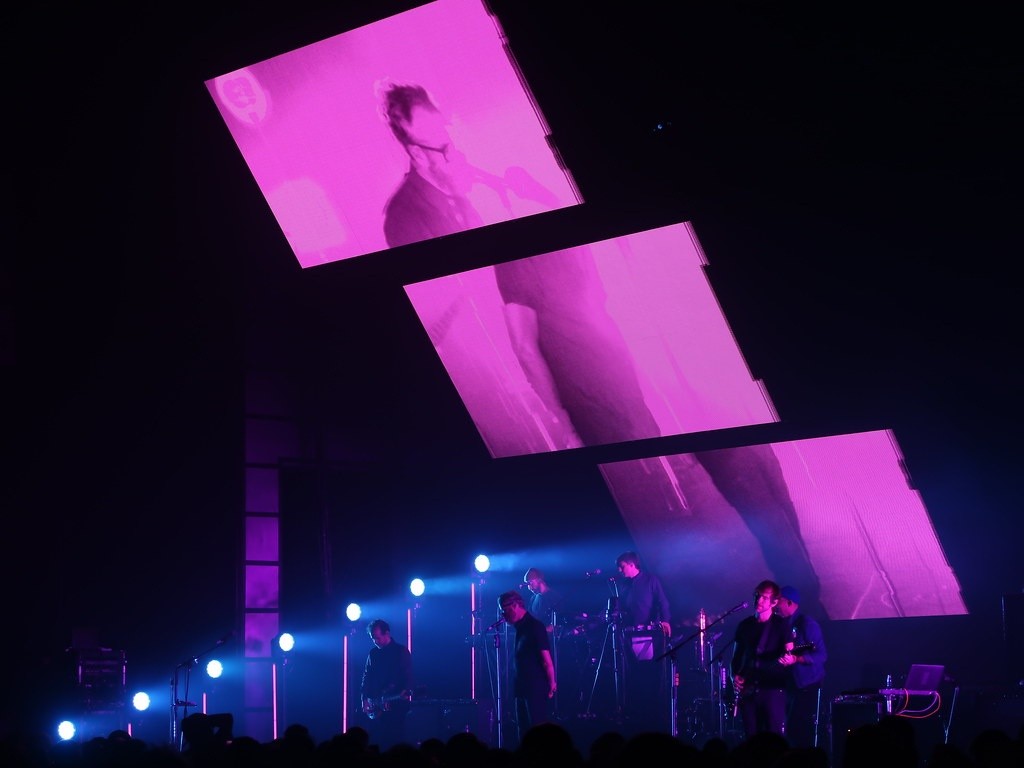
275,633 -> 296,654
408,578 -> 426,597
128,691 -> 151,711
208,660 -> 223,680
51,719 -> 76,742
473,554 -> 490,573
347,603 -> 362,619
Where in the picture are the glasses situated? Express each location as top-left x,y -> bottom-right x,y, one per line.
414,142 -> 459,163
498,602 -> 516,614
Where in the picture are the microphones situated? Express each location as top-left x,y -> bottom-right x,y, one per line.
519,581 -> 533,588
749,612 -> 760,629
452,162 -> 510,191
608,572 -> 625,582
727,601 -> 750,615
585,568 -> 602,577
487,618 -> 506,631
216,630 -> 237,645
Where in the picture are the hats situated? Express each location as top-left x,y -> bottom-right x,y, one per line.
777,585 -> 801,605
523,567 -> 541,581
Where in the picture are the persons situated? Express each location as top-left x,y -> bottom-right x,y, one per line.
772,584 -> 828,749
0,692 -> 1024,767
357,620 -> 417,742
523,565 -> 560,621
382,84 -> 485,250
615,550 -> 674,640
693,442 -> 831,622
730,579 -> 796,740
494,242 -> 661,451
499,591 -> 557,747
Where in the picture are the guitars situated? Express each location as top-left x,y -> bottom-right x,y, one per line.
740,641 -> 819,689
366,690 -> 416,719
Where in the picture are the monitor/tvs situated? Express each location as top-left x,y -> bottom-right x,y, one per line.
591,429 -> 967,625
204,1 -> 587,271
402,218 -> 783,461
